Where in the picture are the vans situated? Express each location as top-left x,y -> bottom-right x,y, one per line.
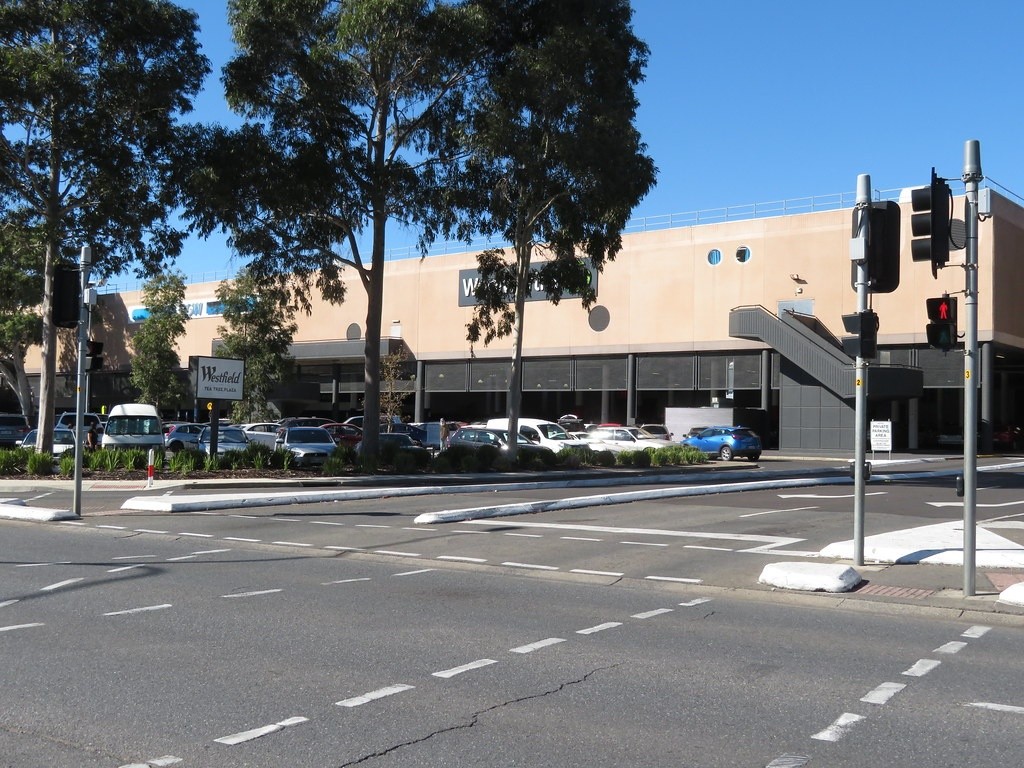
101,403 -> 170,452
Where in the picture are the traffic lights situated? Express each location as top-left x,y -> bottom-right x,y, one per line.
840,313 -> 878,359
911,165 -> 952,280
85,339 -> 105,371
926,295 -> 958,348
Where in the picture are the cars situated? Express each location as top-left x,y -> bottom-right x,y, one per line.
597,423 -> 622,428
678,425 -> 762,461
276,415 -> 593,466
994,424 -> 1024,450
636,424 -> 674,442
163,423 -> 210,452
0,413 -> 33,446
15,428 -> 77,456
274,427 -> 340,467
228,422 -> 282,452
195,426 -> 251,457
590,426 -> 677,451
97,412 -> 109,427
53,411 -> 105,447
683,425 -> 710,439
